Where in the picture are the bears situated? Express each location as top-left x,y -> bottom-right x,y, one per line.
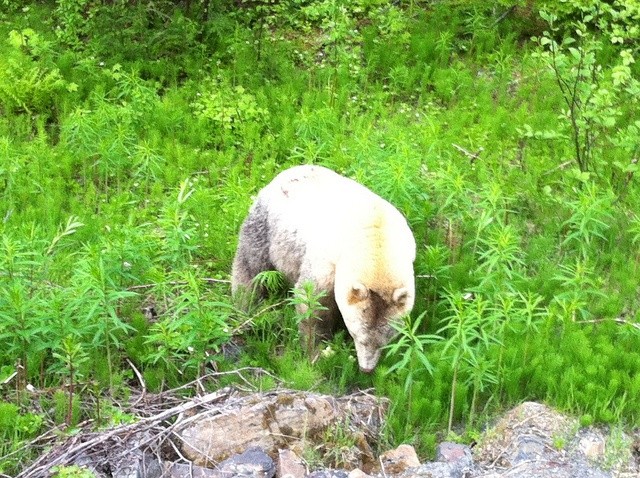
229,164 -> 419,377
